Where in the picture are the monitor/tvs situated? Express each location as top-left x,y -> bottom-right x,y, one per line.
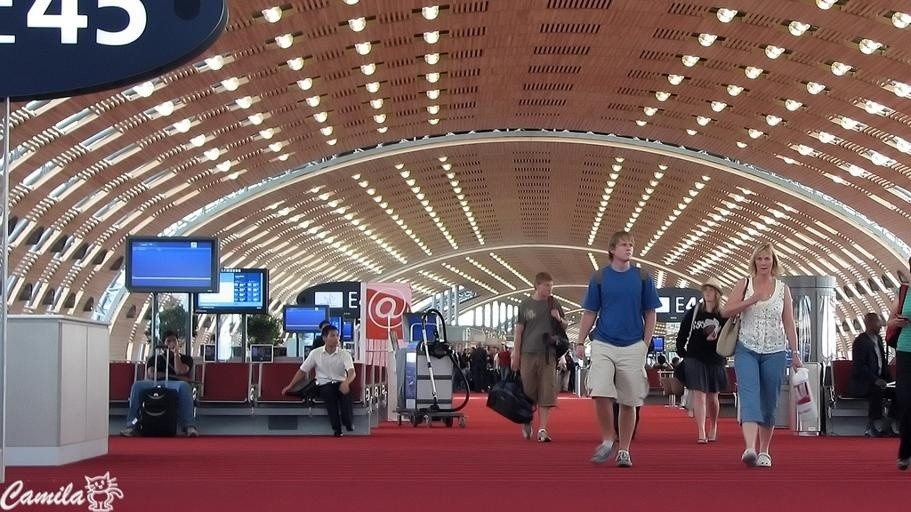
194,268 -> 270,314
651,336 -> 664,352
342,317 -> 353,340
251,344 -> 274,363
283,305 -> 330,333
200,343 -> 205,356
325,316 -> 342,337
204,344 -> 216,362
126,235 -> 219,293
232,346 -> 242,359
274,347 -> 286,358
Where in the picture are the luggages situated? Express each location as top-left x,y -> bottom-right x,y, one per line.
131,346 -> 178,437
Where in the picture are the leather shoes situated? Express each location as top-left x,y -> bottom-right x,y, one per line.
881,426 -> 899,438
334,429 -> 344,436
864,424 -> 881,437
897,457 -> 908,470
346,425 -> 354,431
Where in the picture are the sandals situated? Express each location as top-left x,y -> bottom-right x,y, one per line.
756,452 -> 772,467
741,448 -> 757,465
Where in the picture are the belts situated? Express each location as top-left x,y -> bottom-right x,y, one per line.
332,380 -> 337,384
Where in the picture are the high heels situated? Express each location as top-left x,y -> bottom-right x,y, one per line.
708,437 -> 716,442
697,438 -> 708,443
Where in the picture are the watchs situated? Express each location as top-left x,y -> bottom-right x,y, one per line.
790,349 -> 799,353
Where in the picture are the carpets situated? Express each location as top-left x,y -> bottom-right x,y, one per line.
1,391 -> 911,512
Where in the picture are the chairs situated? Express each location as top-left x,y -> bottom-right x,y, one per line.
109,360 -> 138,416
379,365 -> 388,410
729,366 -> 737,408
885,358 -> 896,407
366,365 -> 376,415
827,359 -> 870,418
144,355 -> 197,420
200,361 -> 252,408
258,363 -> 310,415
136,360 -> 145,381
376,365 -> 382,408
309,363 -> 366,415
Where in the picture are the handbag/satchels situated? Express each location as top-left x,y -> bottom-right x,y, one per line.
486,367 -> 537,424
286,378 -> 320,396
715,278 -> 749,357
543,296 -> 569,365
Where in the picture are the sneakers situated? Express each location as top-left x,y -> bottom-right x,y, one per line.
521,424 -> 533,440
589,440 -> 617,463
186,427 -> 198,437
120,426 -> 140,436
614,448 -> 632,466
537,427 -> 552,442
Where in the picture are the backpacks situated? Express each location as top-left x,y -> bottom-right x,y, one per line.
548,297 -> 570,359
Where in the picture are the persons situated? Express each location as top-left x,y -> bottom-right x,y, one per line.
653,355 -> 674,372
670,357 -> 680,371
559,342 -> 583,395
121,330 -> 201,438
887,256 -> 911,471
672,277 -> 729,444
576,230 -> 662,469
721,242 -> 803,467
851,313 -> 899,439
313,320 -> 331,350
511,272 -> 568,442
496,344 -> 510,380
453,343 -> 495,392
282,325 -> 356,438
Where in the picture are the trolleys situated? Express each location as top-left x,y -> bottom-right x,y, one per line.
392,323 -> 465,428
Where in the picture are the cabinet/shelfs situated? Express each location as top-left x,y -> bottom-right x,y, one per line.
8,315 -> 111,466
